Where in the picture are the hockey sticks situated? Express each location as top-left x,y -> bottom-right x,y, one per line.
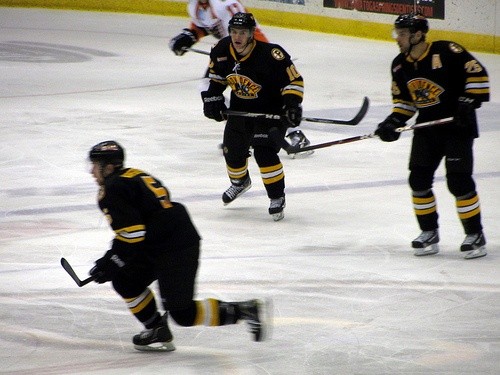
217,95 -> 371,128
60,257 -> 99,286
283,116 -> 453,156
182,46 -> 212,55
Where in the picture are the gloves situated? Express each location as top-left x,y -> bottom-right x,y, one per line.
375,112 -> 411,142
201,91 -> 228,121
169,28 -> 197,56
455,94 -> 481,109
90,249 -> 127,284
282,106 -> 302,127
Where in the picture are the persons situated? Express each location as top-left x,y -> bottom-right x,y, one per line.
200,12 -> 303,221
169,0 -> 315,159
89,140 -> 265,351
374,13 -> 490,259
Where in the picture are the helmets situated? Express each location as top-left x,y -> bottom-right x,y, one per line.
395,13 -> 428,33
228,12 -> 256,33
90,141 -> 124,164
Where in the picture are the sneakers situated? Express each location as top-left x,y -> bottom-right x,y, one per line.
461,230 -> 487,259
288,126 -> 315,159
133,311 -> 176,351
413,229 -> 439,255
269,193 -> 285,221
246,298 -> 275,342
222,169 -> 252,205
